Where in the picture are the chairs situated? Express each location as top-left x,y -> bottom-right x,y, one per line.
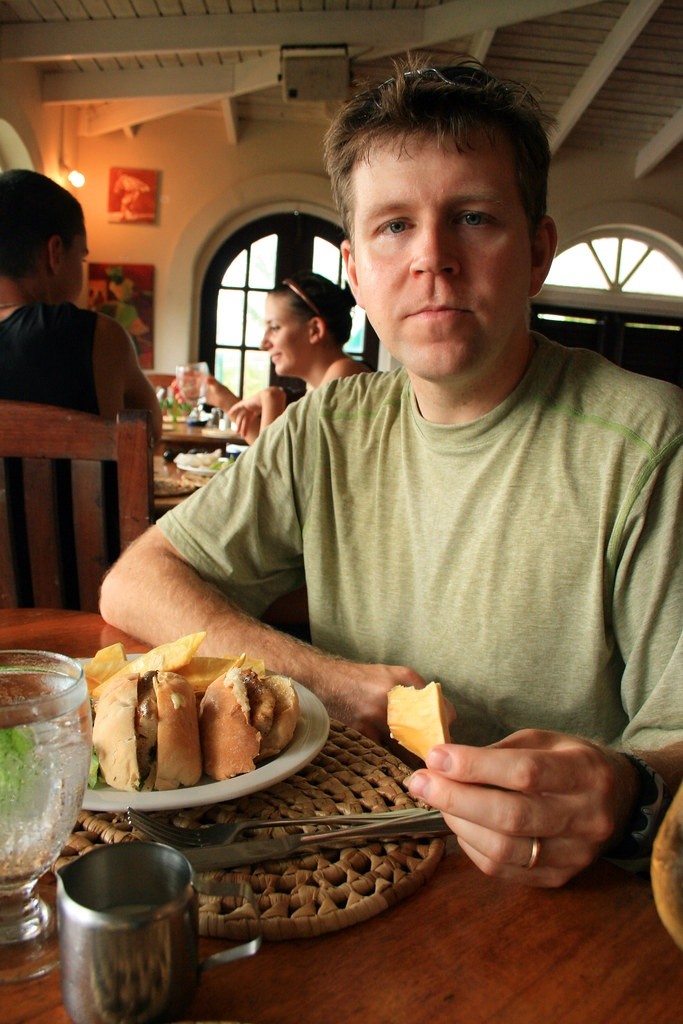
0,387 -> 165,610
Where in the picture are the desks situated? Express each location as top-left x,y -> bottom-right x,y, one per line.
0,603 -> 683,1024
162,423 -> 249,449
153,452 -> 239,511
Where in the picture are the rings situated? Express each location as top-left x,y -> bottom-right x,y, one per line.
521,835 -> 542,872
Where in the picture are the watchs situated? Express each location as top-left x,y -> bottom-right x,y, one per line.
604,753 -> 674,871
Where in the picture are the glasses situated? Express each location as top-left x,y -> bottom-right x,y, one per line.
369,65 -> 509,91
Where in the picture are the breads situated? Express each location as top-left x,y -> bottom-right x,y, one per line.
79,631 -> 267,692
384,681 -> 458,761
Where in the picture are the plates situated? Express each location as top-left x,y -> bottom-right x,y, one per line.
70,653 -> 330,812
175,456 -> 228,475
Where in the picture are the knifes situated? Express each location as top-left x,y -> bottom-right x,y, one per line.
180,809 -> 452,872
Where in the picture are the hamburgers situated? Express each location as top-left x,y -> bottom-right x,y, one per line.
199,665 -> 301,781
92,670 -> 204,794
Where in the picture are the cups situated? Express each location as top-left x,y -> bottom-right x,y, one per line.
0,649 -> 93,983
52,839 -> 265,1024
175,362 -> 214,423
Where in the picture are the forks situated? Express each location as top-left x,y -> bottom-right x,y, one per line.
126,806 -> 431,852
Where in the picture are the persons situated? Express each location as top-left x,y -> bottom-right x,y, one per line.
96,48 -> 683,892
0,168 -> 164,614
